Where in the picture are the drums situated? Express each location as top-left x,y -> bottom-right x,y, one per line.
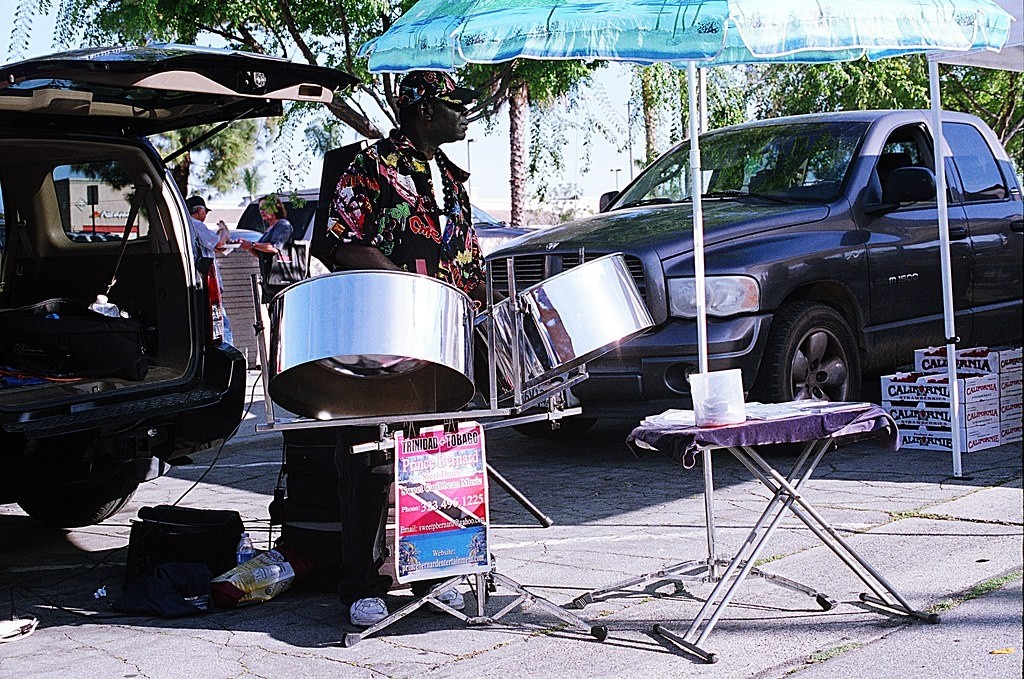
268,266 -> 479,423
473,253 -> 660,405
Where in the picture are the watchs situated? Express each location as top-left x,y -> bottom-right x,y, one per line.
251,242 -> 255,249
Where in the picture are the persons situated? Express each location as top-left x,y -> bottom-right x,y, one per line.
325,70 -> 505,628
237,194 -> 295,306
185,195 -> 235,348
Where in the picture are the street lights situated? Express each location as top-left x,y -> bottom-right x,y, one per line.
467,139 -> 476,201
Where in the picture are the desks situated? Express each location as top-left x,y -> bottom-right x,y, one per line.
627,400 -> 942,664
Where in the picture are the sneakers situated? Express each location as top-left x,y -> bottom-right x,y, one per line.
349,598 -> 388,628
428,581 -> 466,614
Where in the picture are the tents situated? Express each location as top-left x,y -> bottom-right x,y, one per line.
926,0 -> 1024,480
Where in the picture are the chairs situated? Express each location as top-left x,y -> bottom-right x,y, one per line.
877,149 -> 911,198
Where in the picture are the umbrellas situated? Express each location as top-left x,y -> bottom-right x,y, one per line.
355,0 -> 1015,585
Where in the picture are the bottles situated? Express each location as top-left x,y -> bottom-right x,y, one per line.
236,533 -> 255,565
88,294 -> 108,314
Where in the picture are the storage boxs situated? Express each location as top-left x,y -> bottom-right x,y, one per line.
879,347 -> 1023,453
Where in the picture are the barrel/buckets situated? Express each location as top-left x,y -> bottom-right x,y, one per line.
688,369 -> 746,428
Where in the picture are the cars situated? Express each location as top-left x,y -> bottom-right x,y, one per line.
239,191 -> 533,261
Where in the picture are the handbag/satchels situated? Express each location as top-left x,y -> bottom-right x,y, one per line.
266,230 -> 311,287
120,506 -> 245,618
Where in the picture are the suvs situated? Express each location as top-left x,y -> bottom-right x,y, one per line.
1,45 -> 357,531
479,110 -> 1024,442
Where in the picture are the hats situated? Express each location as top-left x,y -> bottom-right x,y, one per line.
396,69 -> 484,108
186,195 -> 213,214
